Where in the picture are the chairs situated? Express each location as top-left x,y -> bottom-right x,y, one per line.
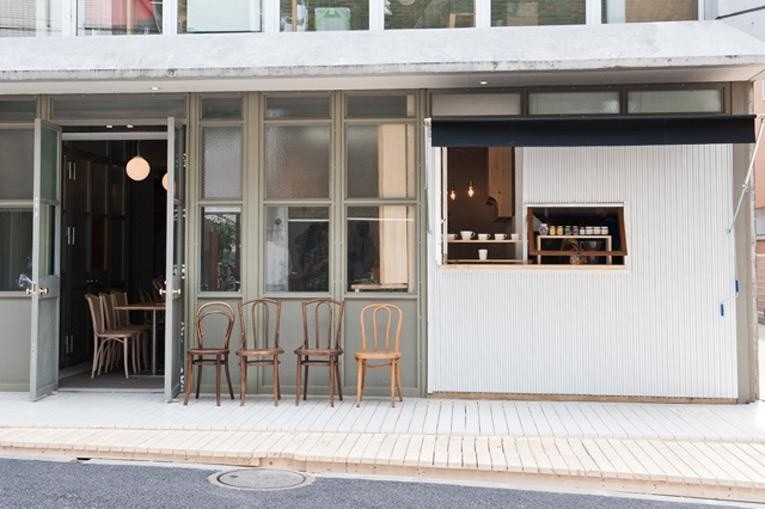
182,297 -> 406,409
82,290 -> 149,380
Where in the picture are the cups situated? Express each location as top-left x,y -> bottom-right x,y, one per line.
448,231 -> 519,240
478,249 -> 488,260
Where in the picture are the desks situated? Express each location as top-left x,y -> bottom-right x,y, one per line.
111,297 -> 167,375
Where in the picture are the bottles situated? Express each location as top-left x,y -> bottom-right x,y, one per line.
539,223 -> 609,235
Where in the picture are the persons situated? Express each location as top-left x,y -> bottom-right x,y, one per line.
466,196 -> 498,227
288,221 -> 377,290
